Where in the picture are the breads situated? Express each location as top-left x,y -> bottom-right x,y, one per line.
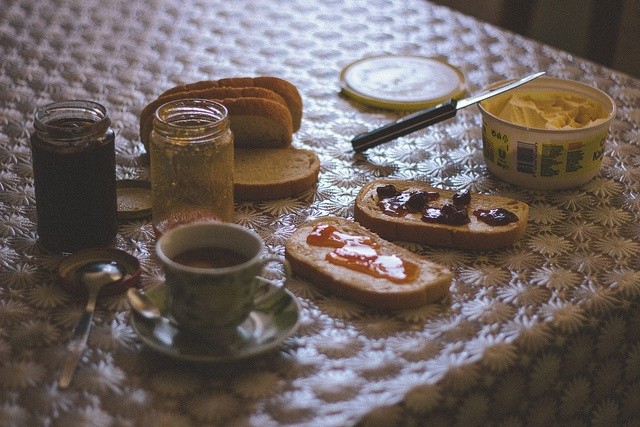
232,146 -> 321,202
169,77 -> 315,132
352,178 -> 533,251
224,95 -> 293,145
138,86 -> 293,143
286,212 -> 455,308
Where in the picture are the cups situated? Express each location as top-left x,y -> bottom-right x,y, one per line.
158,223 -> 288,330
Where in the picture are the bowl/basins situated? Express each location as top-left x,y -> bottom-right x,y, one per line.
477,75 -> 617,190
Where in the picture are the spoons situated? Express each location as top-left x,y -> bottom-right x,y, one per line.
128,286 -> 234,358
50,258 -> 126,388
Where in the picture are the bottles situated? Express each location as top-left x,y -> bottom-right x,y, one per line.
150,99 -> 241,227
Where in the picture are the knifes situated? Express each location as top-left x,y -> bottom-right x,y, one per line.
352,69 -> 546,151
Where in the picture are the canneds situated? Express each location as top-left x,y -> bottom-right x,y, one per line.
144,99 -> 236,233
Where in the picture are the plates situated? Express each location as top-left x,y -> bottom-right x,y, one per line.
133,277 -> 300,362
